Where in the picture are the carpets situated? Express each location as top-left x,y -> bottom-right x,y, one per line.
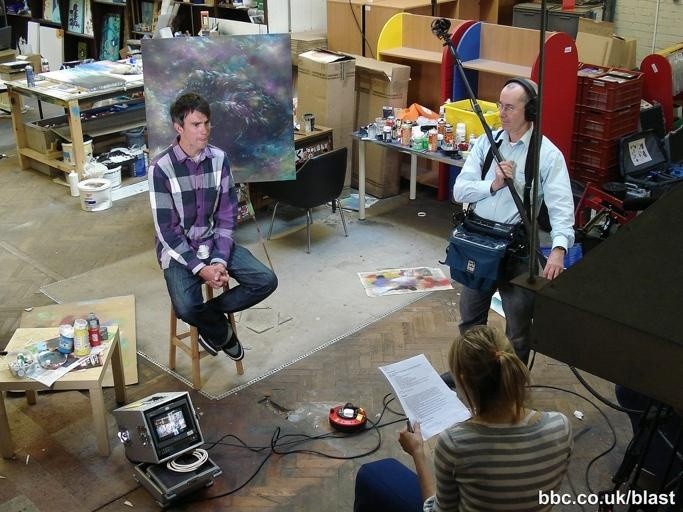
38,210 -> 457,401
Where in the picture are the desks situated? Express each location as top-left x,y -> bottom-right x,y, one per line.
247,122 -> 337,213
0,325 -> 126,457
527,186 -> 682,511
3,54 -> 143,184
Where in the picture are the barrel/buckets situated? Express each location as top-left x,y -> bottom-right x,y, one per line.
103,165 -> 122,188
78,178 -> 113,211
61,139 -> 93,184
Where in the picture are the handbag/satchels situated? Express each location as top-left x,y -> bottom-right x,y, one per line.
446,226 -> 511,294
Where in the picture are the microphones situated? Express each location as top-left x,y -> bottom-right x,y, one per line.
431,17 -> 451,36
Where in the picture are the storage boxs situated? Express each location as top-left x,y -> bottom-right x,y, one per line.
622,36 -> 637,72
575,16 -> 624,68
349,53 -> 410,198
297,47 -> 357,199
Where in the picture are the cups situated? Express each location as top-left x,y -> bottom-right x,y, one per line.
303,113 -> 315,134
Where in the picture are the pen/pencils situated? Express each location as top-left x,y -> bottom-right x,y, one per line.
407,420 -> 412,432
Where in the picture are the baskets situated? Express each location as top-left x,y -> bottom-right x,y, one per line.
567,62 -> 646,185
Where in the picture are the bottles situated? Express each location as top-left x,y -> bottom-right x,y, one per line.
68,170 -> 80,198
41,58 -> 50,74
74,319 -> 90,356
368,115 -> 476,156
58,324 -> 74,354
88,313 -> 101,347
24,63 -> 35,89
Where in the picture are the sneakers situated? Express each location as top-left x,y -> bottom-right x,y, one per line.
198,333 -> 244,362
439,371 -> 456,386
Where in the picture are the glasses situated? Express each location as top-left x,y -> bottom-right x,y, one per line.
495,101 -> 514,113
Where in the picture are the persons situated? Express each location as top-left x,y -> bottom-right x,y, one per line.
438,79 -> 576,389
352,325 -> 575,512
147,92 -> 277,362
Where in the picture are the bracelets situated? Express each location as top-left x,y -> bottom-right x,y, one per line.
552,246 -> 566,256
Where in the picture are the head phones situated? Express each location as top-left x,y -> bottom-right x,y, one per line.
502,77 -> 538,122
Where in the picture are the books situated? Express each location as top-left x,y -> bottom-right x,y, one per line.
576,67 -> 638,86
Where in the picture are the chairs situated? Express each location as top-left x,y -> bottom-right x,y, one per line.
264,147 -> 348,253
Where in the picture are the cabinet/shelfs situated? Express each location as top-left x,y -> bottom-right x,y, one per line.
546,4 -> 598,39
375,12 -> 477,199
327,0 -> 518,61
0,0 -> 130,61
171,0 -> 249,37
448,21 -> 577,227
24,97 -> 146,176
513,2 -> 555,31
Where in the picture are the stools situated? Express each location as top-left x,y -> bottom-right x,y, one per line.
168,278 -> 244,390
